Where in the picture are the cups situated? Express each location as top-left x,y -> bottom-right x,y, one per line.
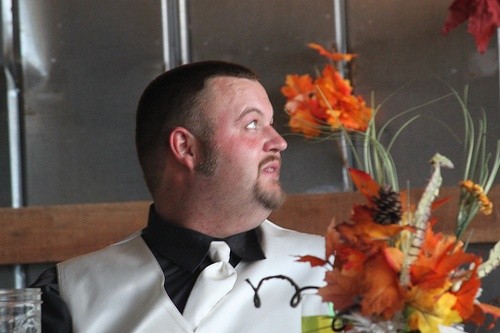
1,287 -> 44,333
301,291 -> 345,333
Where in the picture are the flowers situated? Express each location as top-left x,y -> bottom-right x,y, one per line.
276,38 -> 500,333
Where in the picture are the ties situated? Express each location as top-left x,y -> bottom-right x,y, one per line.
183,238 -> 240,333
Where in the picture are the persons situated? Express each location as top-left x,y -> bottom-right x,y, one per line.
28,60 -> 471,333
